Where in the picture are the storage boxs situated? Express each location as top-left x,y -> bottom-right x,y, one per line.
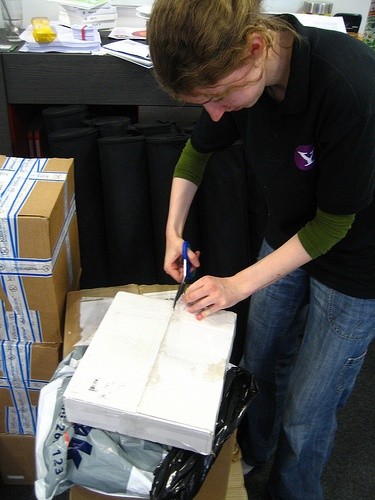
0,153 -> 250,500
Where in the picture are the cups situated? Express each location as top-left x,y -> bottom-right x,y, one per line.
1,0 -> 22,43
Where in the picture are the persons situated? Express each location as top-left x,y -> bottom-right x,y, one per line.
148,0 -> 373,500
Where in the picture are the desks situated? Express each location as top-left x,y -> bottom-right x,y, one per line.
0,26 -> 202,153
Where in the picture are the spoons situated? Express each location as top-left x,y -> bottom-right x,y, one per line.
1,0 -> 20,36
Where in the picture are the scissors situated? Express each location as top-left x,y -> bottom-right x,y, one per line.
172,241 -> 199,310
